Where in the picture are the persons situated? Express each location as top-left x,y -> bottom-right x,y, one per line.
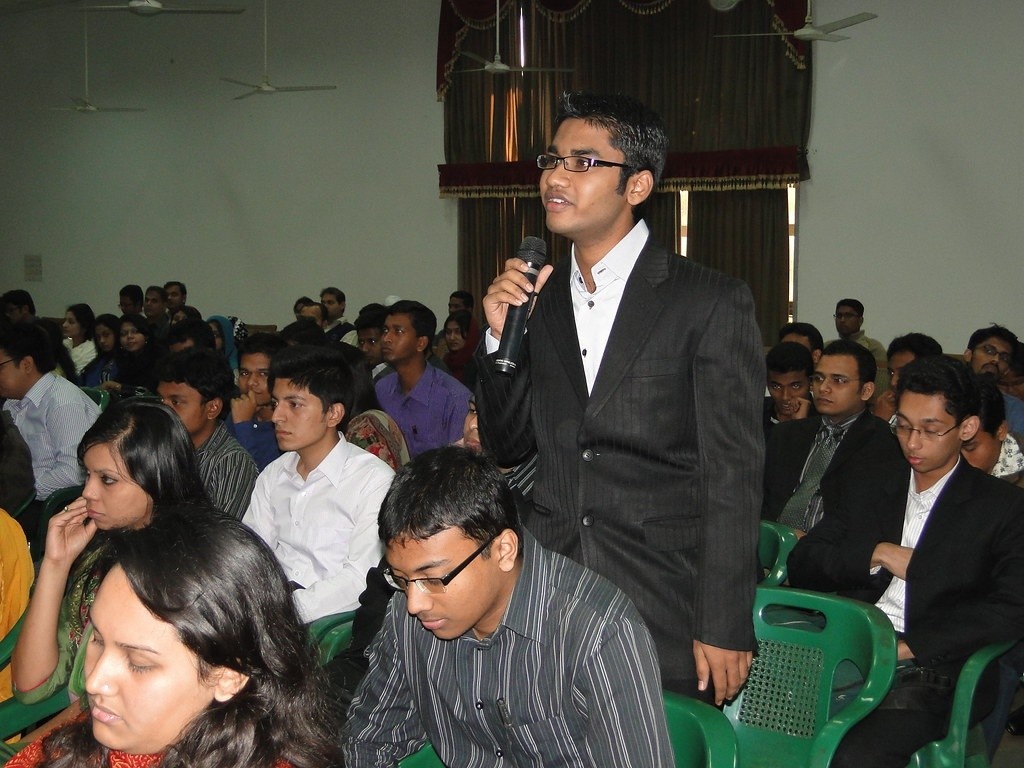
0,280 -> 1024,768
469,91 -> 763,711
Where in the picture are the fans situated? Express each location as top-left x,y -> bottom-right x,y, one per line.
68,0 -> 246,16
39,0 -> 149,114
449,0 -> 580,76
711,0 -> 878,44
216,0 -> 337,103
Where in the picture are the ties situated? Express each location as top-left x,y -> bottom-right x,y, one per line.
779,425 -> 843,535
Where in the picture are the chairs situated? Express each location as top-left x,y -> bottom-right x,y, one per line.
0,385 -> 1022,768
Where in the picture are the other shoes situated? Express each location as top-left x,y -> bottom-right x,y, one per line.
1005,705 -> 1024,736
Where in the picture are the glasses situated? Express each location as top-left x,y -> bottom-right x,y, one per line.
118,303 -> 138,308
888,413 -> 971,441
972,343 -> 1013,364
808,372 -> 866,385
535,153 -> 637,173
833,312 -> 858,319
382,526 -> 507,595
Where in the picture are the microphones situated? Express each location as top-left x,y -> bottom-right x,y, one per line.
493,235 -> 546,377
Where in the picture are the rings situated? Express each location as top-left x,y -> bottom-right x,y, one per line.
64,506 -> 68,512
